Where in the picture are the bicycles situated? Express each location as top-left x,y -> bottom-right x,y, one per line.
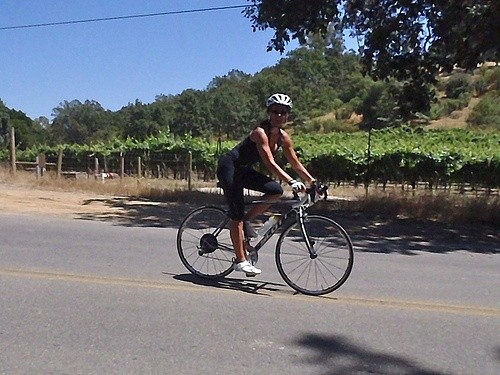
176,181 -> 354,296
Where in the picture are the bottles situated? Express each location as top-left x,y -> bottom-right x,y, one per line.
259,215 -> 279,236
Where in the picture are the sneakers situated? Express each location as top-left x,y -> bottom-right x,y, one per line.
233,260 -> 261,273
246,221 -> 258,237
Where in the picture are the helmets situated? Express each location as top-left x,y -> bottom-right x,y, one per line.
266,93 -> 292,109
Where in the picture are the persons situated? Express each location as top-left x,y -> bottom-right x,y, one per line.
215,93 -> 329,275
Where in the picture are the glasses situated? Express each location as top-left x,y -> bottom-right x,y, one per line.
270,109 -> 287,115
